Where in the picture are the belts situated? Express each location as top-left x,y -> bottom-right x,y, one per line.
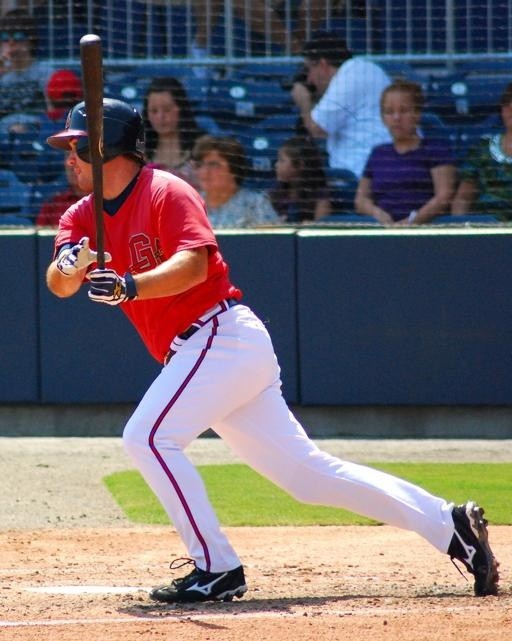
165,297 -> 240,365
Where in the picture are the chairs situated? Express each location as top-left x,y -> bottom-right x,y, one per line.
1,61 -> 512,226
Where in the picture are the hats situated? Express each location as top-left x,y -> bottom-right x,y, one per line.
47,71 -> 83,102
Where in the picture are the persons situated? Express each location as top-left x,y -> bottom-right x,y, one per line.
1,0 -> 365,225
44,97 -> 500,611
294,33 -> 395,178
354,84 -> 512,222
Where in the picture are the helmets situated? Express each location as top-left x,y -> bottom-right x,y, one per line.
46,97 -> 145,163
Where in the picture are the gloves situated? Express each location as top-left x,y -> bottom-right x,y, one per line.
85,268 -> 138,306
56,237 -> 112,277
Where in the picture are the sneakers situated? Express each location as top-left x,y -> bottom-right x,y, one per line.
149,558 -> 247,602
448,501 -> 499,596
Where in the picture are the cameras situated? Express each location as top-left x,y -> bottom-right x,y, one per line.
280,73 -> 316,93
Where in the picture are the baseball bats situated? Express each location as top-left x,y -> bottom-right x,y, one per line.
79,33 -> 105,272
